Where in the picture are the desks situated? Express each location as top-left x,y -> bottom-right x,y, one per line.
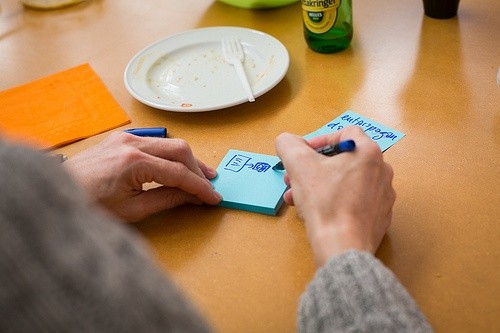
1,0 -> 500,332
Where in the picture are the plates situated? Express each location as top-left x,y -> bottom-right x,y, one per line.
123,26 -> 290,112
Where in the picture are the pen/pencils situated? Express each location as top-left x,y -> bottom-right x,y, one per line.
272,140 -> 355,170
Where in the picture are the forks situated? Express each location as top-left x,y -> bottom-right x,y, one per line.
219,35 -> 256,102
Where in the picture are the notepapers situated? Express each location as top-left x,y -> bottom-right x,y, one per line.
203,148 -> 289,216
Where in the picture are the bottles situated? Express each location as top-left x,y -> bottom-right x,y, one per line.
301,0 -> 354,54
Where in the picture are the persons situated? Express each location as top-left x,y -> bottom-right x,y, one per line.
0,124 -> 434,333
